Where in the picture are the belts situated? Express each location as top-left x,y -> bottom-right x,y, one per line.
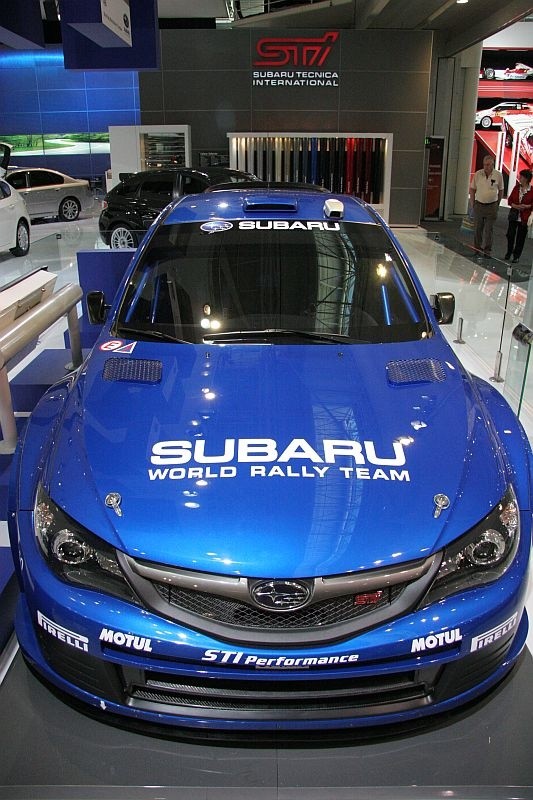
477,201 -> 497,205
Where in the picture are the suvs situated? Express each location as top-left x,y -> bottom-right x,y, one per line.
97,166 -> 265,251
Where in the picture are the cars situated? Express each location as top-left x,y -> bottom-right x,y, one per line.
0,179 -> 533,734
476,101 -> 533,130
480,59 -> 533,82
0,167 -> 90,222
0,175 -> 32,257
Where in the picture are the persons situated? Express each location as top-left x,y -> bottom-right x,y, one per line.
469,155 -> 505,259
504,169 -> 533,264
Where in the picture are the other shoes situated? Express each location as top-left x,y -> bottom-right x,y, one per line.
512,257 -> 519,263
505,251 -> 512,260
483,252 -> 492,258
474,250 -> 481,257
